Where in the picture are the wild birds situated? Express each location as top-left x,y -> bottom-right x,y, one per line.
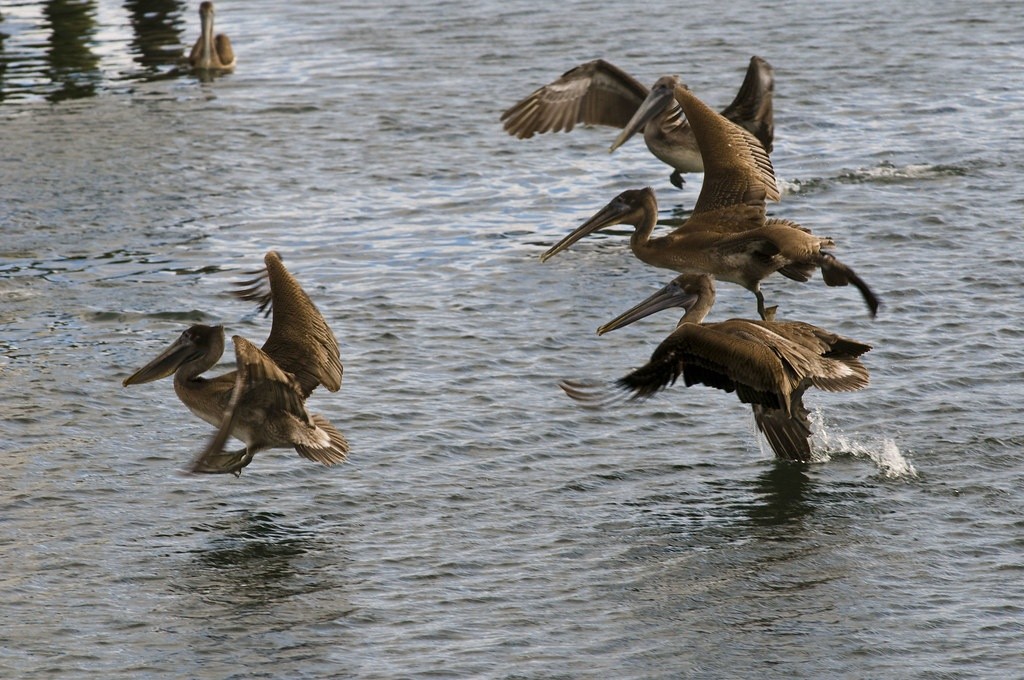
120,249 -> 351,476
187,1 -> 235,71
501,55 -> 881,464
